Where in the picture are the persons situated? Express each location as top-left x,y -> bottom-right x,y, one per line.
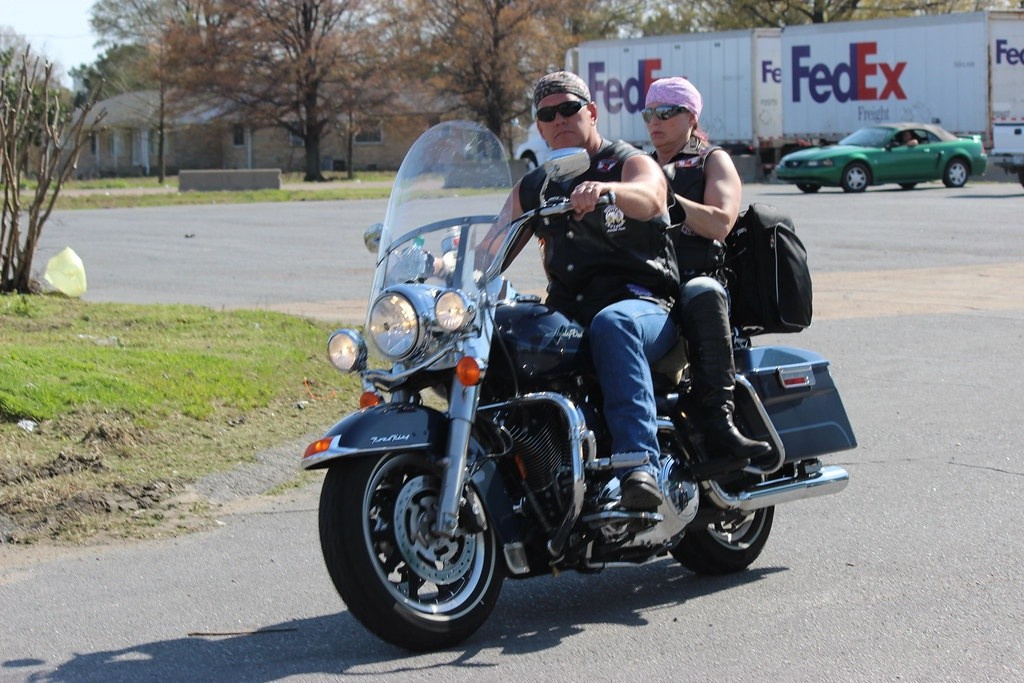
406,72 -> 681,512
641,76 -> 772,463
893,131 -> 918,146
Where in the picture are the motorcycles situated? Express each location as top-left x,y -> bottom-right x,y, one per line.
300,119 -> 860,652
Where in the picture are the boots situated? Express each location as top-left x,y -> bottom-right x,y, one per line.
681,289 -> 772,459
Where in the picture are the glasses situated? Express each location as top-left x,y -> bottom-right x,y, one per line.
537,100 -> 588,122
642,105 -> 689,123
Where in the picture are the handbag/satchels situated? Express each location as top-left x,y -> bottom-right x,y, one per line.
722,203 -> 812,335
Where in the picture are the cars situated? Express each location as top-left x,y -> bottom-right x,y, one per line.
774,120 -> 990,193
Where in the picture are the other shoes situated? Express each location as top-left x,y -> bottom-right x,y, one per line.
620,469 -> 662,525
